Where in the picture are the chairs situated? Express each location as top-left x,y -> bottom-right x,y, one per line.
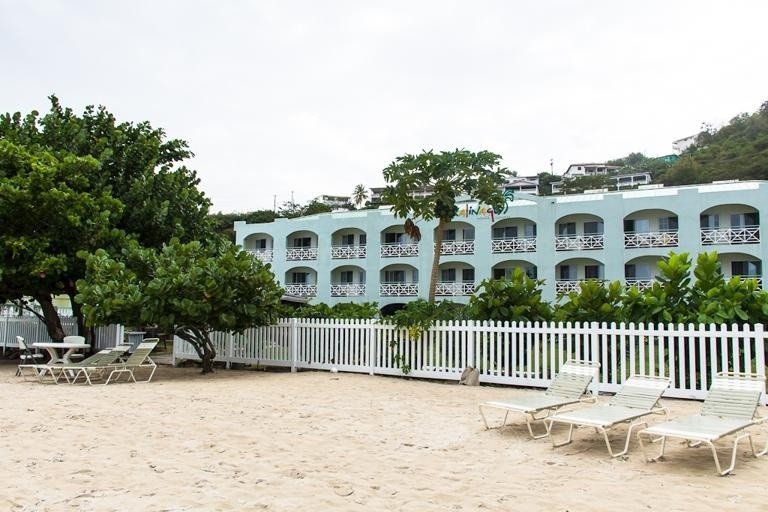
14,335 -> 160,386
478,358 -> 768,477
